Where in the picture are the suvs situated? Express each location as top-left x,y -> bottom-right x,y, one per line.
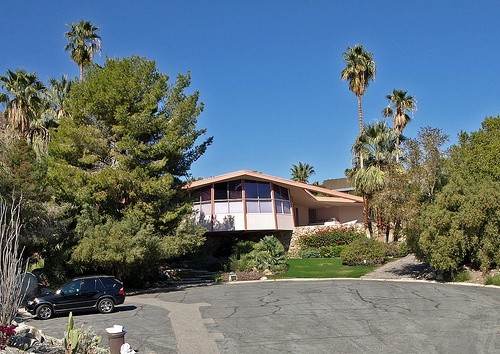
25,275 -> 125,320
